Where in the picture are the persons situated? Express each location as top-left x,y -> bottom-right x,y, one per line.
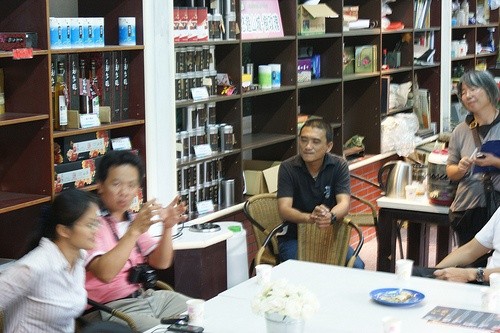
446,71 -> 500,268
85,150 -> 192,332
277,119 -> 365,268
413,208 -> 500,285
0,189 -> 131,333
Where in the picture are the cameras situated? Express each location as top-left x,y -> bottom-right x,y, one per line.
127,262 -> 158,285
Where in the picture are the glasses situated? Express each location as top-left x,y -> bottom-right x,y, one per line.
62,219 -> 100,230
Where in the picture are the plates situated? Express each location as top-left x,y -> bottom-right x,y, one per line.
370,287 -> 426,306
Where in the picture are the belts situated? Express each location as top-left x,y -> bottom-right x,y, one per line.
128,290 -> 142,298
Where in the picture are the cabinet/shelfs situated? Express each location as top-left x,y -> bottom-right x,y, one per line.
0,0 -> 148,266
172,0 -> 442,227
451,0 -> 500,132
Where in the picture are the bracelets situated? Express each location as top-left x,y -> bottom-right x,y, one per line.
477,268 -> 484,281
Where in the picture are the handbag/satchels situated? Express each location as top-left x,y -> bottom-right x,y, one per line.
483,178 -> 500,220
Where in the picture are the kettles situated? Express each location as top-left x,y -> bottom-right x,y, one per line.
378,159 -> 414,199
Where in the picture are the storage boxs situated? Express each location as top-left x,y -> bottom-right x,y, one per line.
297,0 -> 339,36
242,159 -> 282,196
1,32 -> 38,53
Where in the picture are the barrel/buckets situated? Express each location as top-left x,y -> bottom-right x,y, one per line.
427,162 -> 458,200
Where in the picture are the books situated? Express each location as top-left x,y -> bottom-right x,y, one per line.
415,30 -> 436,65
50,49 -> 129,128
414,0 -> 430,28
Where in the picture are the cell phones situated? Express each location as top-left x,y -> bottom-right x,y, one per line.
161,314 -> 204,333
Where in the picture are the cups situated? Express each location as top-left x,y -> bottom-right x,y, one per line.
266,312 -> 303,333
255,264 -> 272,285
259,65 -> 272,90
268,64 -> 281,89
394,259 -> 415,279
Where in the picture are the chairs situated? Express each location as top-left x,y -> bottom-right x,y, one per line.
0,258 -> 141,333
244,172 -> 380,279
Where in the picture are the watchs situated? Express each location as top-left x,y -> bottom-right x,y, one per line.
330,212 -> 337,224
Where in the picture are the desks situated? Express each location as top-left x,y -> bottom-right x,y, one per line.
376,186 -> 454,279
166,256 -> 499,333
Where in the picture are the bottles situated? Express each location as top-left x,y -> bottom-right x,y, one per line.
428,149 -> 450,165
221,178 -> 235,208
382,49 -> 387,65
